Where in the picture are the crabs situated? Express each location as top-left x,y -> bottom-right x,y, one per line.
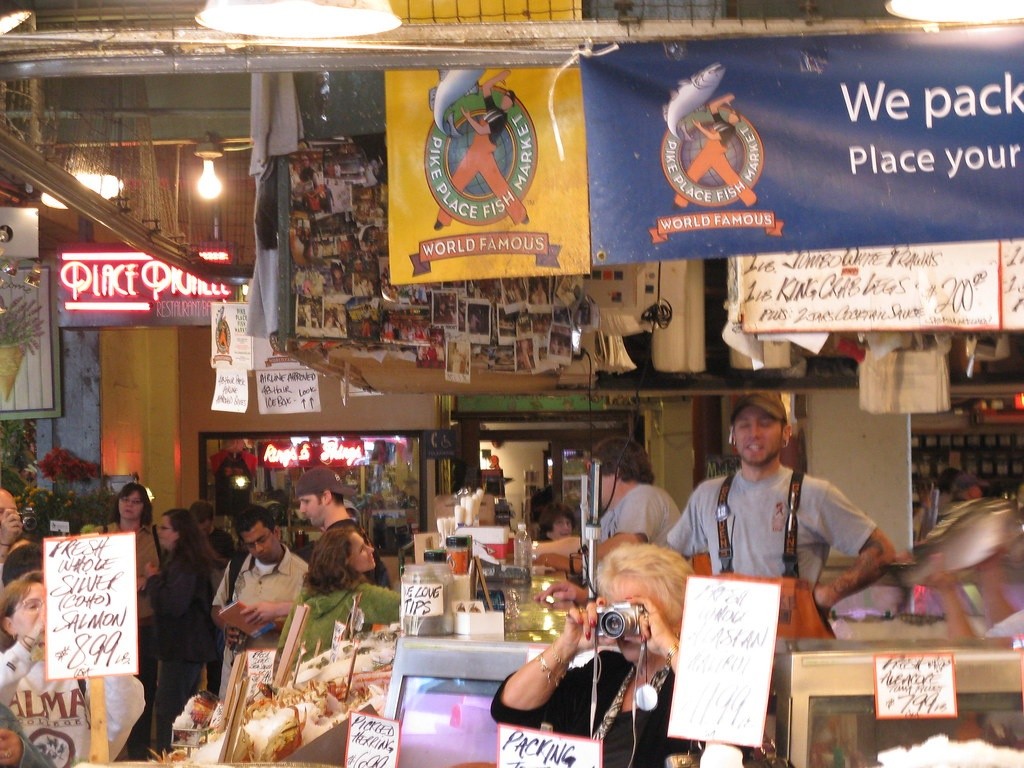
143,676 -> 375,768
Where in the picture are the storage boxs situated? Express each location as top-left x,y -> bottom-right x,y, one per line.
456,524 -> 517,560
450,611 -> 506,635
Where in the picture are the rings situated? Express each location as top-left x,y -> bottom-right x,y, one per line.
4,749 -> 12,758
545,557 -> 548,562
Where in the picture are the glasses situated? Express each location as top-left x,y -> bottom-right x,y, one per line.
14,598 -> 42,612
244,531 -> 271,548
120,496 -> 142,506
161,525 -> 171,531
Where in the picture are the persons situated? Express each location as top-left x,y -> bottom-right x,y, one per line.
927,506 -> 1024,636
0,465 -> 400,760
490,541 -> 755,768
914,467 -> 1024,541
534,393 -> 897,638
531,435 -> 693,576
0,571 -> 146,768
0,702 -> 56,768
290,147 -> 592,376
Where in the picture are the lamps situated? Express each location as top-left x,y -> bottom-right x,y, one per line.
40,145 -> 123,210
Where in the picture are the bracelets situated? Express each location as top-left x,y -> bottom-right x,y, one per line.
22,635 -> 36,650
551,641 -> 576,665
537,653 -> 568,686
665,641 -> 679,667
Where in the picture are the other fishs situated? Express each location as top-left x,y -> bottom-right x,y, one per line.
876,495 -> 1023,590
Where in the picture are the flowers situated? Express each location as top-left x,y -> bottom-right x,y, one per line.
12,486 -> 82,517
38,446 -> 102,484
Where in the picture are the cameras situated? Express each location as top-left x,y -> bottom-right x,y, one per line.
18,508 -> 39,531
593,602 -> 647,638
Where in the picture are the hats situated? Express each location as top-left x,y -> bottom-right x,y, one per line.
296,465 -> 356,497
955,471 -> 991,491
730,391 -> 784,430
343,499 -> 362,527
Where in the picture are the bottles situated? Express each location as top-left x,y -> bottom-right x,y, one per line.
423,550 -> 451,584
444,536 -> 468,576
513,523 -> 533,570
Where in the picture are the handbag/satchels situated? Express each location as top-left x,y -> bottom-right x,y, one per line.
213,625 -> 228,677
